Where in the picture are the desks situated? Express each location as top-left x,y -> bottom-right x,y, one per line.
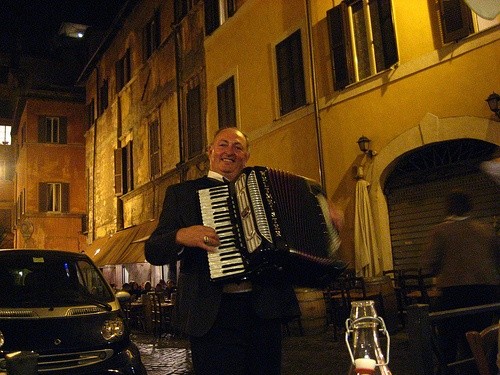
131,303 -> 171,308
354,277 -> 389,332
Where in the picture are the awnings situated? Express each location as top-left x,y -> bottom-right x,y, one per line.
86,220 -> 159,268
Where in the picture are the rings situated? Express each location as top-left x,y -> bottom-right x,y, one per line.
204,235 -> 208,242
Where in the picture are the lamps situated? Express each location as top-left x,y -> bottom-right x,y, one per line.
357,135 -> 376,157
484,91 -> 500,118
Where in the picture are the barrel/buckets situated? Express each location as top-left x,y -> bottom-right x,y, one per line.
295,287 -> 327,335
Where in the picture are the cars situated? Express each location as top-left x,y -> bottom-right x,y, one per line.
0,248 -> 148,375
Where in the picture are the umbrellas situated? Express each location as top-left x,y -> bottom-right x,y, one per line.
354,180 -> 380,278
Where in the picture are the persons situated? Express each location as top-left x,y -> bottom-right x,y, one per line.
124,280 -> 176,302
420,193 -> 500,375
142,127 -> 344,374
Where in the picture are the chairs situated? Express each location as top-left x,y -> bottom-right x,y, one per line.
323,268 -> 500,375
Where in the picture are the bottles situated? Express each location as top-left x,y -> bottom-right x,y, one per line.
348,300 -> 390,375
